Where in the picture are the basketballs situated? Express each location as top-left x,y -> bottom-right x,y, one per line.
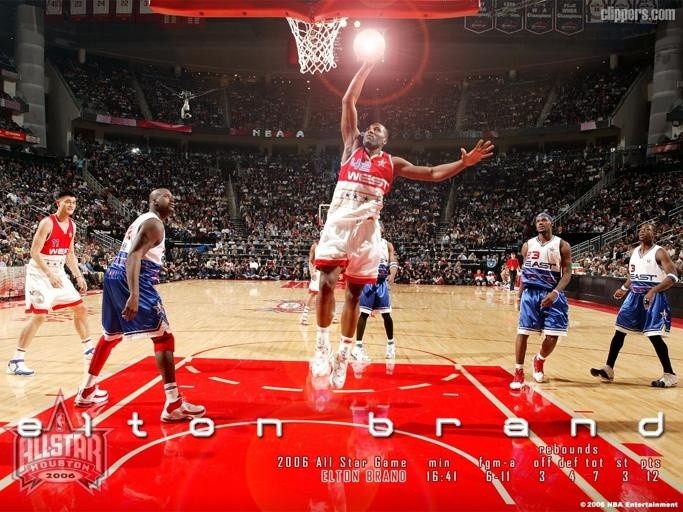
354,29 -> 384,63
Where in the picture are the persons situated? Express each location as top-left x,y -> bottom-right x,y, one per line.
6,191 -> 95,375
312,54 -> 495,390
1,46 -> 682,291
352,221 -> 399,362
590,223 -> 679,388
74,188 -> 207,425
510,212 -> 571,390
301,236 -> 338,325
341,400 -> 390,484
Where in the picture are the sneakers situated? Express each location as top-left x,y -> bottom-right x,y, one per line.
651,372 -> 678,387
301,315 -> 308,325
510,368 -> 526,390
329,353 -> 349,388
386,343 -> 395,359
74,384 -> 108,408
532,355 -> 545,382
161,392 -> 206,423
312,343 -> 333,377
332,314 -> 338,324
7,359 -> 34,376
590,365 -> 614,383
351,347 -> 362,361
84,348 -> 96,361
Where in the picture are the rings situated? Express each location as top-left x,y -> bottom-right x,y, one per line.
644,299 -> 650,304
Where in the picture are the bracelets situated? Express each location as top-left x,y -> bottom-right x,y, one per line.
553,288 -> 560,297
515,297 -> 522,301
